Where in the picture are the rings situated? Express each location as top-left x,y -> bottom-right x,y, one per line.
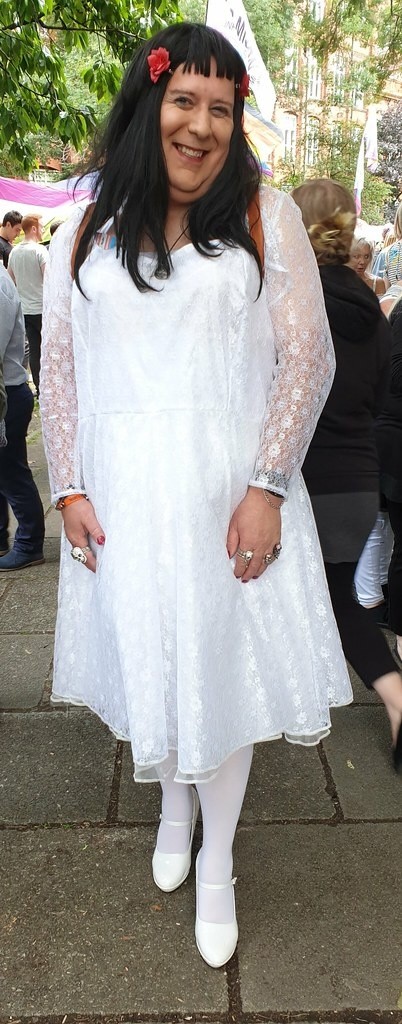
263,554 -> 273,564
70,547 -> 91,563
238,551 -> 253,560
273,544 -> 282,559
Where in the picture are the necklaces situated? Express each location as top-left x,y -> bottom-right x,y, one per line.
146,223 -> 190,280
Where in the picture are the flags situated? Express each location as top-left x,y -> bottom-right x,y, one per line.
356,111 -> 379,217
242,99 -> 284,178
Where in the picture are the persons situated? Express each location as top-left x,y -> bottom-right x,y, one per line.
354,280 -> 402,663
371,202 -> 402,284
347,236 -> 385,295
0,210 -> 23,270
46,221 -> 62,251
0,262 -> 46,571
7,214 -> 49,403
39,22 -> 336,968
288,179 -> 402,763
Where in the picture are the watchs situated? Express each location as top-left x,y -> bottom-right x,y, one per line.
256,471 -> 287,498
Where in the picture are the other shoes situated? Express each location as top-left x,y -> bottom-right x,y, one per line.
195,845 -> 239,969
394,725 -> 402,773
152,786 -> 200,893
0,550 -> 45,571
0,540 -> 8,556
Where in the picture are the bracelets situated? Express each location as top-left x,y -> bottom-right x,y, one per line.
263,490 -> 284,510
54,486 -> 89,511
